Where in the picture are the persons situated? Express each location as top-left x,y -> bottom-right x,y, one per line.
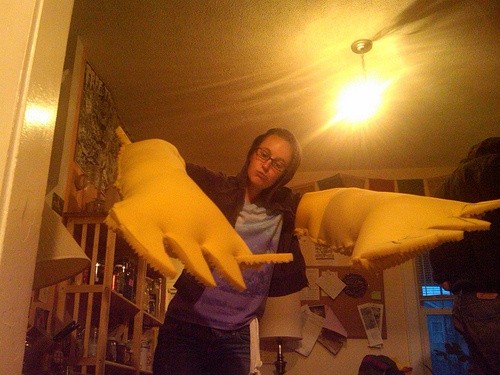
103,128 -> 500,375
428,135 -> 500,375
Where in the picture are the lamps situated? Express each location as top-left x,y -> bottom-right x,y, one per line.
259,292 -> 303,375
32,204 -> 92,291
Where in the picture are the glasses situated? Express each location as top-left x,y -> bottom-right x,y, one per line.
254,146 -> 288,172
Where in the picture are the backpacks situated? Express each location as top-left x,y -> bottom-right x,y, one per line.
358,354 -> 404,375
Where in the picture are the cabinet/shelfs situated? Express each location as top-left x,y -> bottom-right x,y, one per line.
40,211 -> 168,375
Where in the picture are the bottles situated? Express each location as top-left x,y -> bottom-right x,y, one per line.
96,257 -> 135,299
